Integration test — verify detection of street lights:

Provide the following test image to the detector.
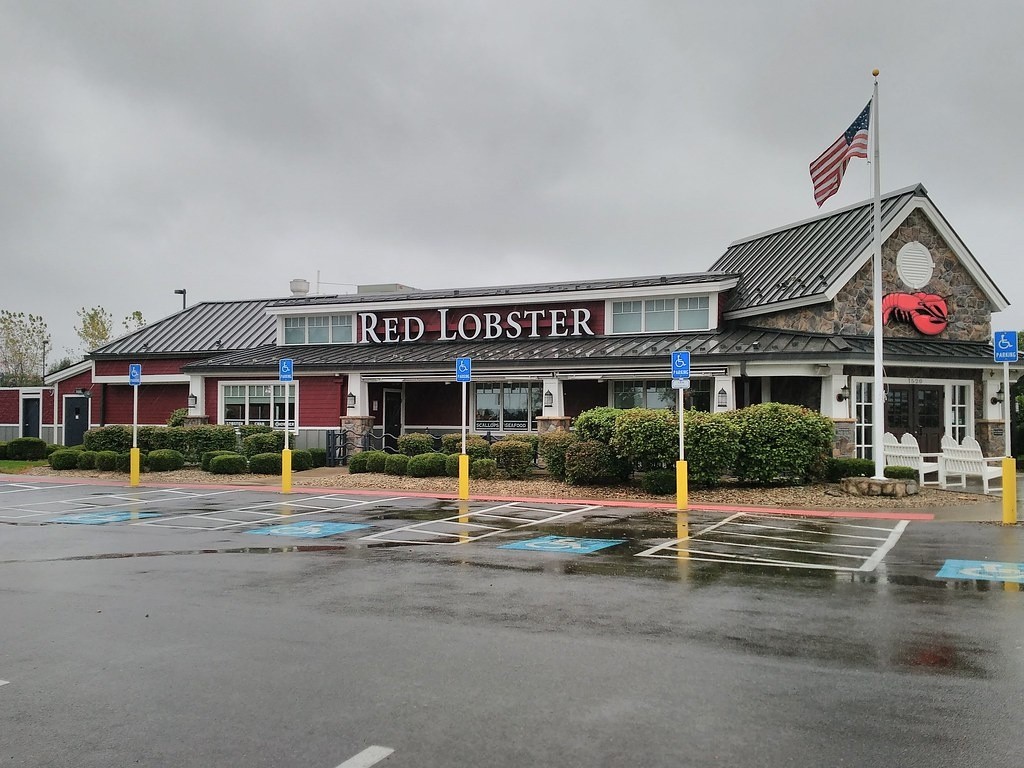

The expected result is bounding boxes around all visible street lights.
[174,289,186,309]
[42,340,50,377]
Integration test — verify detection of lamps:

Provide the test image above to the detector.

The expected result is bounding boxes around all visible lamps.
[187,393,197,408]
[836,385,850,403]
[991,389,1004,405]
[718,388,727,407]
[347,392,356,408]
[544,389,553,407]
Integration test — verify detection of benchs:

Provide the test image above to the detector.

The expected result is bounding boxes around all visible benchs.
[941,434,1007,495]
[884,432,941,487]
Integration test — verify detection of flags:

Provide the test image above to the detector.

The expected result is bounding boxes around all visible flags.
[809,96,872,208]
